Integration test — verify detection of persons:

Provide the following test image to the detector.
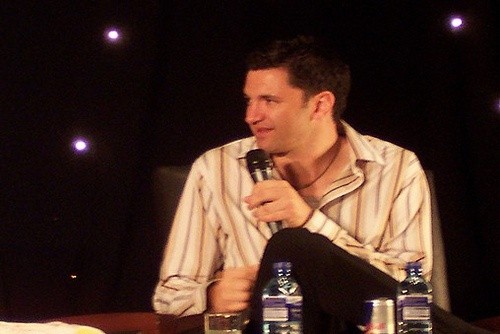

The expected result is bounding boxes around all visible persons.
[152,32,432,318]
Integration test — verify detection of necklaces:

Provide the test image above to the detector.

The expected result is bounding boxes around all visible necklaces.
[271,138,342,189]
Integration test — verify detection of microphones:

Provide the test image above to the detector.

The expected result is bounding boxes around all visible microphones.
[245,149,283,235]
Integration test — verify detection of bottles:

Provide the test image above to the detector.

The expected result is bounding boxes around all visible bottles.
[262,262,303,334]
[398,262,433,334]
